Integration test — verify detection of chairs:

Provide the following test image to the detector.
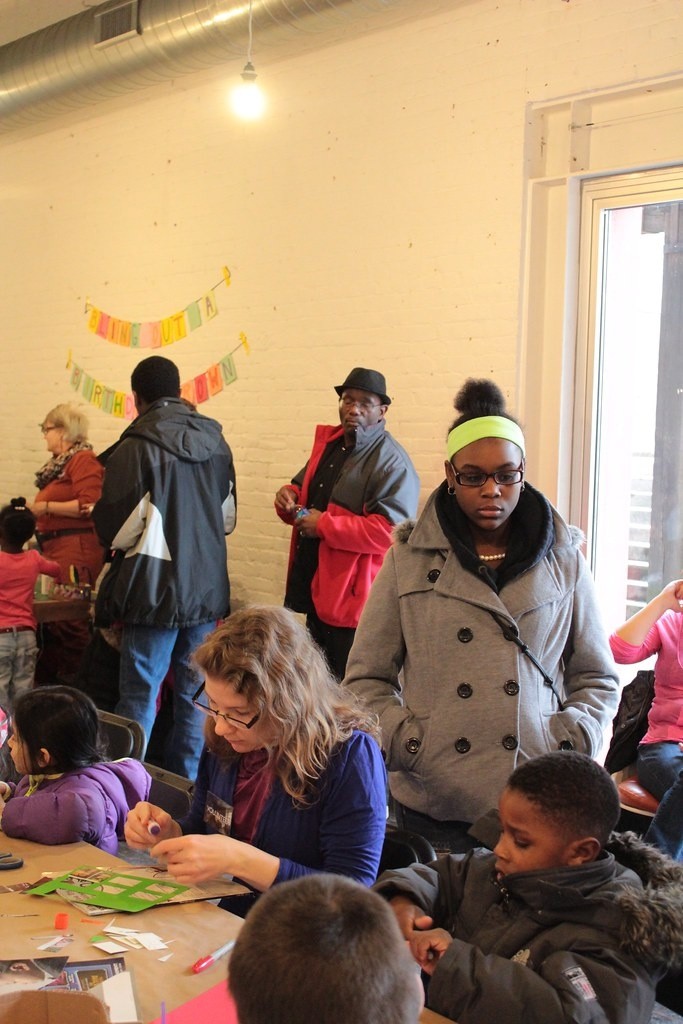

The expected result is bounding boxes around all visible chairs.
[374,826,439,888]
[615,670,658,827]
[135,760,197,828]
[95,711,145,762]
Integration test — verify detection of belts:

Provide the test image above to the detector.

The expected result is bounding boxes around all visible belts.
[36,527,95,541]
[0,626,34,633]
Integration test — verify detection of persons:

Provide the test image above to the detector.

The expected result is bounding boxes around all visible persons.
[228,872,424,1024]
[340,380,623,867]
[371,749,683,1023]
[0,497,59,786]
[273,369,420,686]
[0,685,151,855]
[24,404,106,689]
[123,606,387,922]
[90,357,236,832]
[608,580,683,866]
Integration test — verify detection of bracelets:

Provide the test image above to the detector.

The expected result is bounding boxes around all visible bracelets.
[45,501,48,515]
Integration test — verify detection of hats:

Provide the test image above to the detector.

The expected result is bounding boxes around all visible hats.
[333,367,391,406]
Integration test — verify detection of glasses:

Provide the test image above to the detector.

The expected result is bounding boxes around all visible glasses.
[448,458,525,487]
[339,396,382,411]
[191,680,262,729]
[41,426,64,435]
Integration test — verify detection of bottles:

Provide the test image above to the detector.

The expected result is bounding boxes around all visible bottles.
[289,502,309,524]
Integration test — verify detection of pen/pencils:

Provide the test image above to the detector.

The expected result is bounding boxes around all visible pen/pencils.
[191,940,236,974]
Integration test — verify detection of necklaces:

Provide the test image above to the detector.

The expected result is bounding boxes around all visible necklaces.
[478,553,505,561]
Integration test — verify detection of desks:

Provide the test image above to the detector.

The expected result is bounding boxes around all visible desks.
[2,824,455,1024]
[30,591,92,662]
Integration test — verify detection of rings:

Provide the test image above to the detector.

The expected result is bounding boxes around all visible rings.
[299,530,305,537]
